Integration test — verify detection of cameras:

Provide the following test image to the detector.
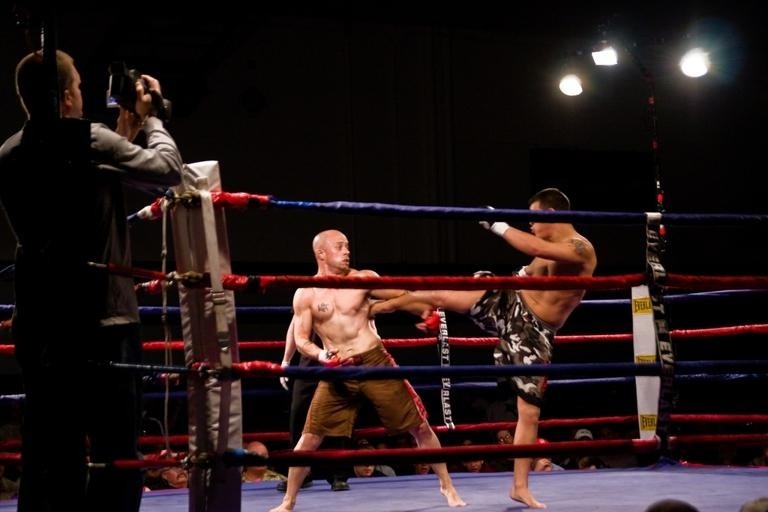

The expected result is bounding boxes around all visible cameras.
[103,68,173,118]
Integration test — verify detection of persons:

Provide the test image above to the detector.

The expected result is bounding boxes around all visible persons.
[645,497,698,511]
[276,312,352,491]
[266,229,470,512]
[1,429,611,495]
[366,185,599,509]
[739,495,768,512]
[0,45,185,512]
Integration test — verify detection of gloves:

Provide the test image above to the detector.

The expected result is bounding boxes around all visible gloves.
[280,360,290,391]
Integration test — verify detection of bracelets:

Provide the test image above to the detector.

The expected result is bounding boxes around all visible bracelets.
[489,220,510,237]
[518,264,528,278]
[318,349,328,364]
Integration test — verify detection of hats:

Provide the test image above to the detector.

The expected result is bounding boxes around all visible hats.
[575,428,594,440]
